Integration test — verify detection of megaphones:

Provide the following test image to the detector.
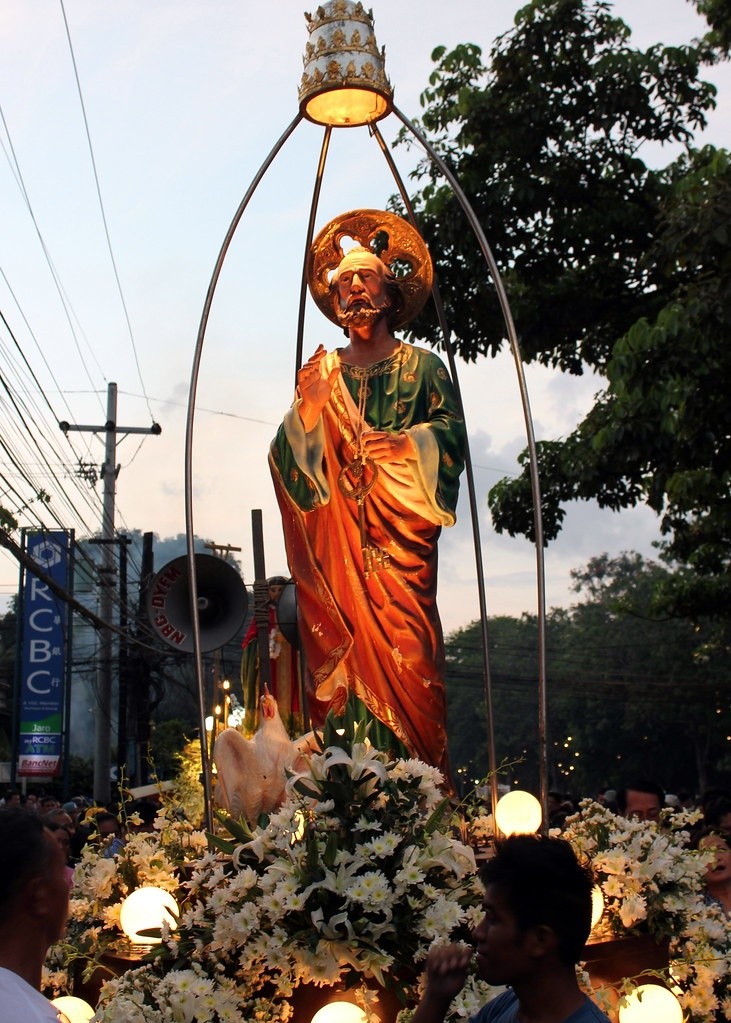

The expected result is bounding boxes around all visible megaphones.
[148,553,248,655]
[276,578,297,649]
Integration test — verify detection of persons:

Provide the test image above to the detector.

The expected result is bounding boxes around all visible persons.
[268,248,465,837]
[4,789,161,871]
[0,804,96,1023]
[549,780,731,922]
[240,577,286,730]
[411,834,612,1023]
